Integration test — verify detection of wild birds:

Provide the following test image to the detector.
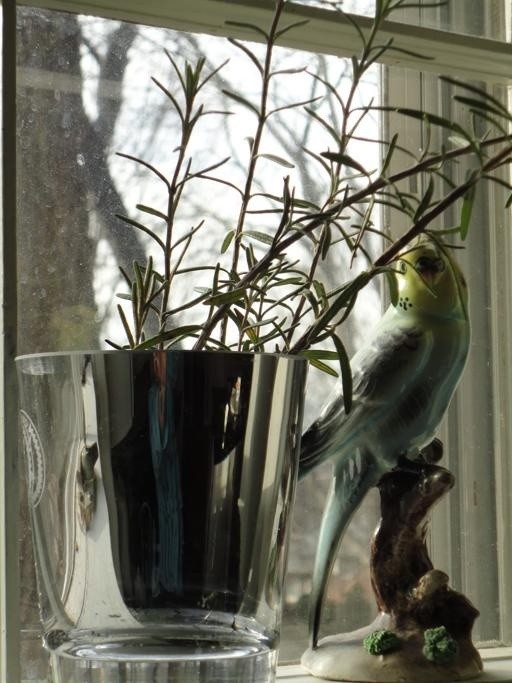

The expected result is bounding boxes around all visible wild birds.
[296,233,471,651]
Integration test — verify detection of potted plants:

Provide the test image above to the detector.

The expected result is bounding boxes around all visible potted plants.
[4,1,512,680]
